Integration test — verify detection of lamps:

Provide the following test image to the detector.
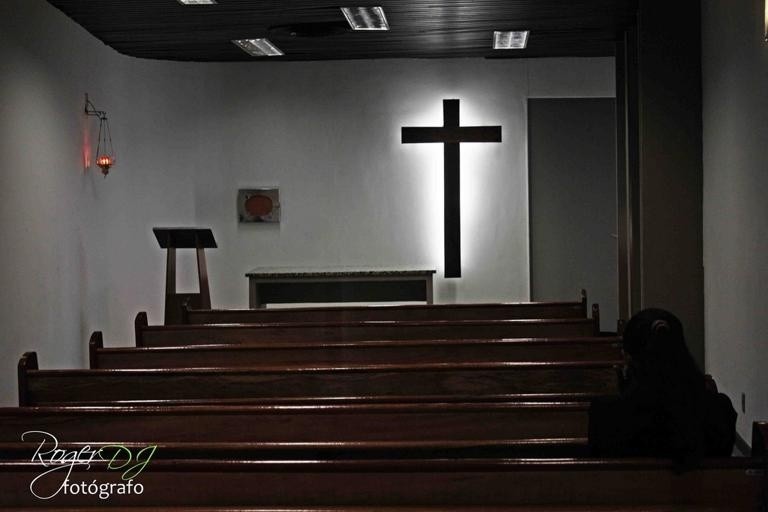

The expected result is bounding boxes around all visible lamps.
[80,89,117,180]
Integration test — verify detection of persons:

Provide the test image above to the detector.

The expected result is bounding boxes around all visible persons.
[588,307,738,464]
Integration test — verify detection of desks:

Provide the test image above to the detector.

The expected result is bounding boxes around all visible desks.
[245,268,436,311]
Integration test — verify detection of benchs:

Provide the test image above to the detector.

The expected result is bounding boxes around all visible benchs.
[0,288,763,507]
